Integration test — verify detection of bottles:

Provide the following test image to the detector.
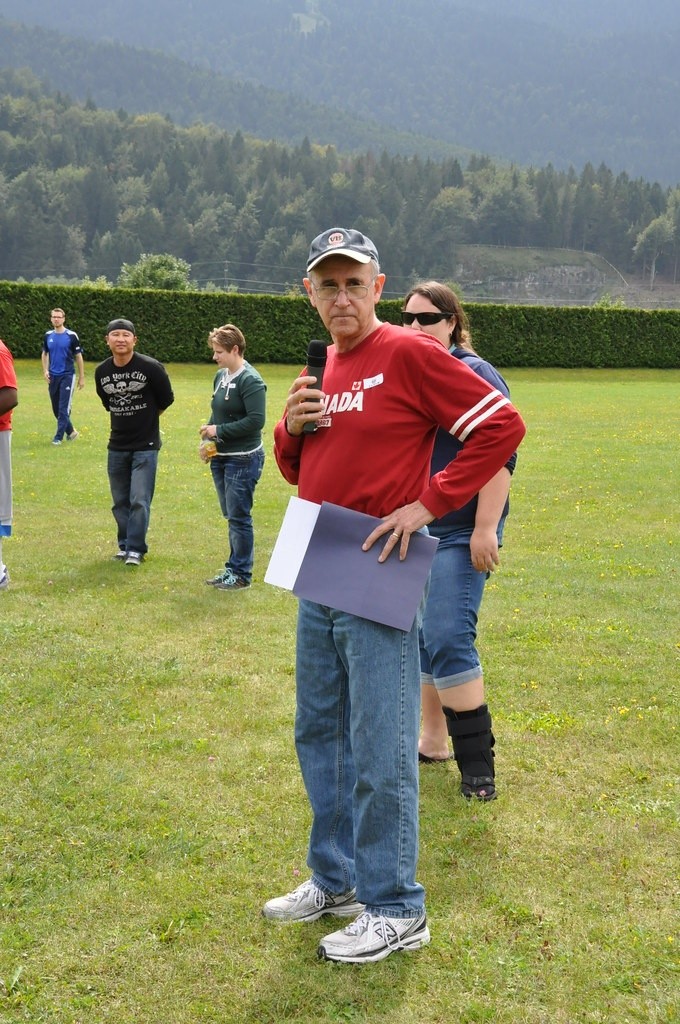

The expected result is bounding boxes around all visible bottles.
[200,419,217,457]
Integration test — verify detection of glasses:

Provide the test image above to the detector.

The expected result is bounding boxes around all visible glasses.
[51,315,66,320]
[401,310,455,325]
[308,278,377,301]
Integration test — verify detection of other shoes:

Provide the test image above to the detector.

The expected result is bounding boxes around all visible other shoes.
[67,429,78,441]
[52,438,61,444]
[0,564,9,589]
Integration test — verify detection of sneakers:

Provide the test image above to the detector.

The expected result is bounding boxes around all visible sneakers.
[318,907,431,964]
[262,876,368,924]
[215,571,251,592]
[116,551,126,561]
[126,553,141,566]
[206,569,232,585]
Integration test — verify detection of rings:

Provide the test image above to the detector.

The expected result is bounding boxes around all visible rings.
[393,533,400,538]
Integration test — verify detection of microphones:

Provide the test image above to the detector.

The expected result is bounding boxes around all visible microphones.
[303,340,327,433]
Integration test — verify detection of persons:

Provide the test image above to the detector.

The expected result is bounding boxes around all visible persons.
[94,319,175,566]
[260,223,525,961]
[400,281,517,802]
[0,338,18,589]
[41,308,85,445]
[198,323,267,592]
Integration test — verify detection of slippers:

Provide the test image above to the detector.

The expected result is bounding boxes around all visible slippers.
[416,752,454,763]
[457,771,498,801]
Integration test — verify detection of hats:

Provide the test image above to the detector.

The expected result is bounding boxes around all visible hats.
[107,319,135,336]
[305,227,379,274]
